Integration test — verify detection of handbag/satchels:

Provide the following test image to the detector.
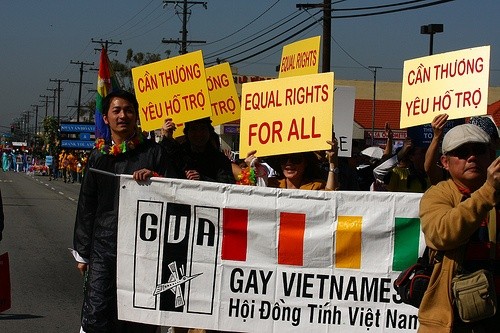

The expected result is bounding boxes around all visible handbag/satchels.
[450,268,496,324]
[394,264,432,309]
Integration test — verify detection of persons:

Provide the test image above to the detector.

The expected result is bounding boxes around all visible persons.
[158,114,500,193]
[417,124,500,333]
[67,90,166,333]
[0,140,89,183]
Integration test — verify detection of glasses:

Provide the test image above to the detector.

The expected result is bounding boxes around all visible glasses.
[280,155,305,166]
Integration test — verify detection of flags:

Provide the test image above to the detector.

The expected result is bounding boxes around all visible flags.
[95,48,112,142]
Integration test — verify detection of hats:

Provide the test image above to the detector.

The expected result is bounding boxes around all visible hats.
[441,124,492,155]
